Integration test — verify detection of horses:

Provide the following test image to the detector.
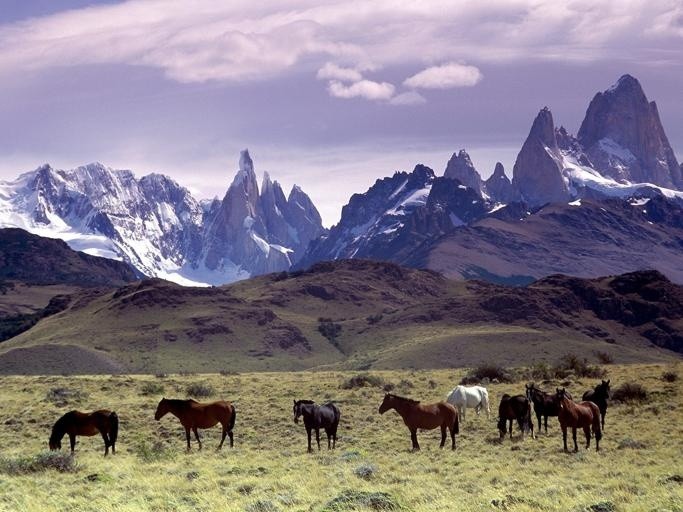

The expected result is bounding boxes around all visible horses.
[47,408,119,458]
[447,384,490,421]
[153,396,237,451]
[378,391,460,451]
[292,398,340,451]
[495,379,612,453]
